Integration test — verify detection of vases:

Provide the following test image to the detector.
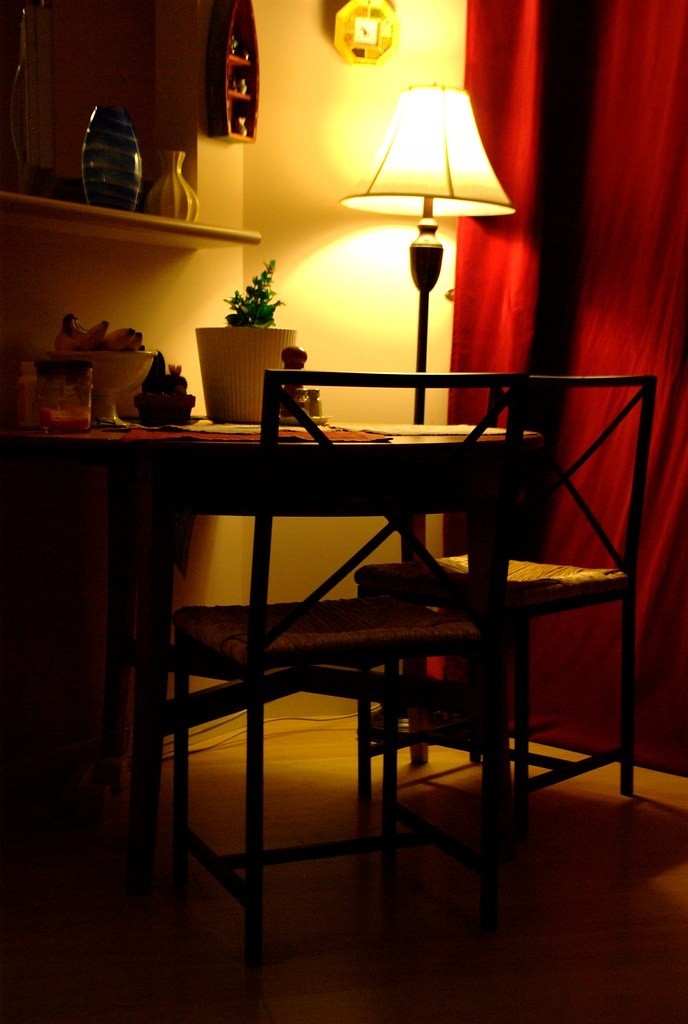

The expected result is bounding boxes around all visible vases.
[146,151,199,224]
[81,106,142,211]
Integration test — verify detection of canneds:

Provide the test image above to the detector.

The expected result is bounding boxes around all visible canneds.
[34,359,93,432]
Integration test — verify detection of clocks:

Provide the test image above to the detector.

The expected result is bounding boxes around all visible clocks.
[334,0,399,66]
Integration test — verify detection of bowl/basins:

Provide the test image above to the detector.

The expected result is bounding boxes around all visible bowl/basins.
[49,351,156,426]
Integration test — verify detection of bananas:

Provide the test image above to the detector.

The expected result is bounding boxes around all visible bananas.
[54,312,143,351]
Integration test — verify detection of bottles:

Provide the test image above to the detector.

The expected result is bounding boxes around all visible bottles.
[146,151,198,222]
[82,105,140,212]
[35,361,92,433]
[17,361,35,427]
[295,388,324,417]
[277,346,307,416]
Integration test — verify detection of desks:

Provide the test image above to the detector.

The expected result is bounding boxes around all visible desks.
[0,419,543,898]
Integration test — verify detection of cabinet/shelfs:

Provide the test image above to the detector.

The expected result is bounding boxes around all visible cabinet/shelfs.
[206,0,260,141]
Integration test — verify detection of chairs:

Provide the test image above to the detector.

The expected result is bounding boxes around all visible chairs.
[355,375,659,844]
[171,368,530,970]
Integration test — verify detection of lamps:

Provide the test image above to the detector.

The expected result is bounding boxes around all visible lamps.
[338,84,517,425]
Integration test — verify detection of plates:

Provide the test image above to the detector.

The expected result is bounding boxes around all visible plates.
[278,416,330,431]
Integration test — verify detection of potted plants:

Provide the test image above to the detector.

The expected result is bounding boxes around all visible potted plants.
[195,260,298,424]
